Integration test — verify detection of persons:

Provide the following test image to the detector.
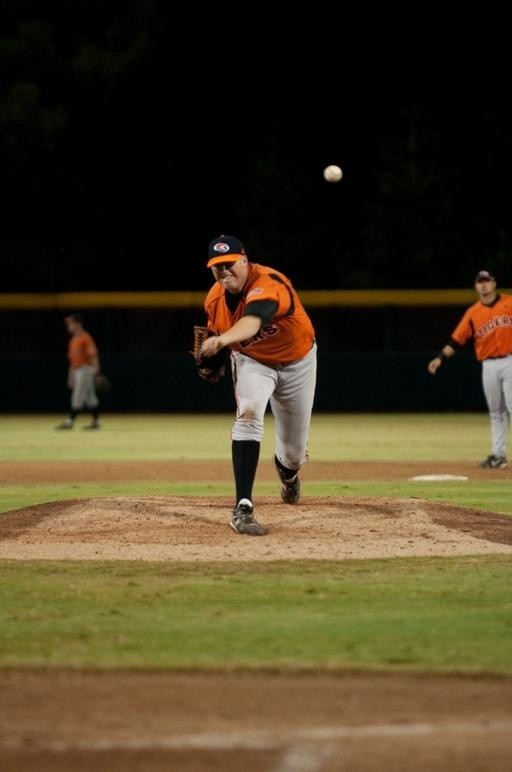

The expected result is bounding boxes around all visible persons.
[56,315,103,429]
[199,236,317,536]
[428,270,512,469]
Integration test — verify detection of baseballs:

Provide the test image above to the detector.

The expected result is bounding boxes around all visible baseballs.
[324,165,342,181]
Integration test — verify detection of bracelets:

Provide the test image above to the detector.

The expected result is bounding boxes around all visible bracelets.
[438,352,448,362]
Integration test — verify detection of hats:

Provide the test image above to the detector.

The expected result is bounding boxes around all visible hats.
[206,234,245,268]
[475,270,495,281]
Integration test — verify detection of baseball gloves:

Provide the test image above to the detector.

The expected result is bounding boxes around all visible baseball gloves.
[193,326,232,383]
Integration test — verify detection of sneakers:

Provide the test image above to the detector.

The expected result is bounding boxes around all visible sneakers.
[274,456,300,504]
[480,455,507,470]
[229,497,264,535]
